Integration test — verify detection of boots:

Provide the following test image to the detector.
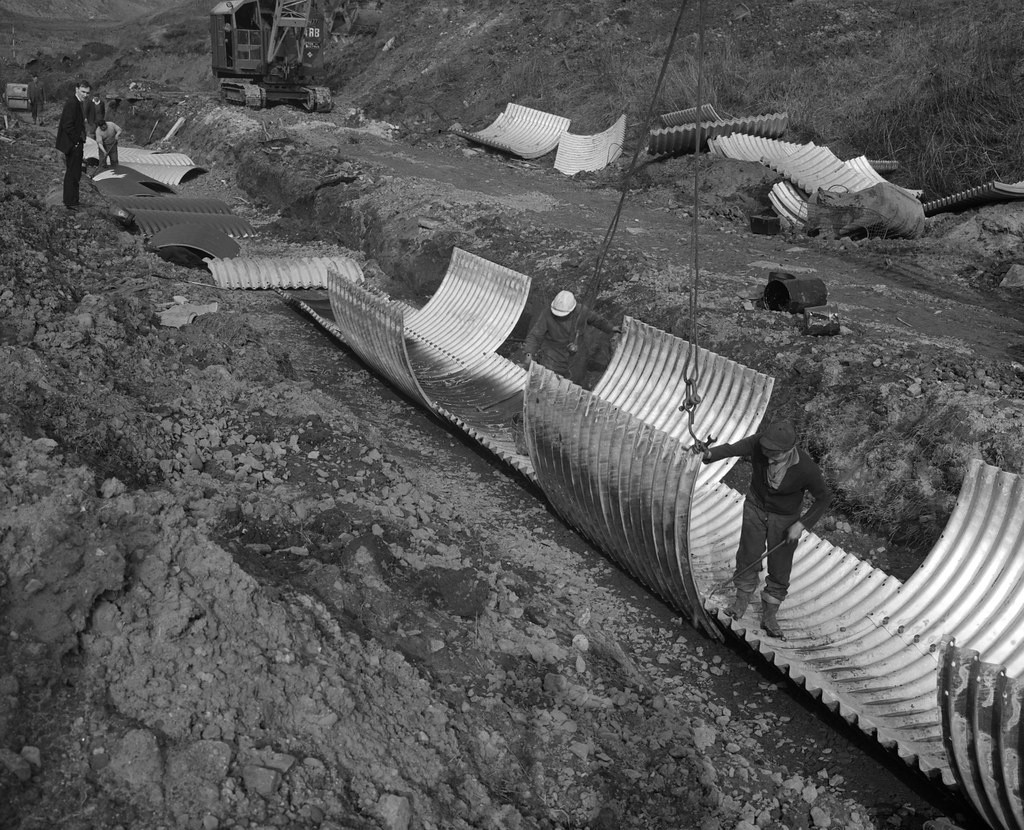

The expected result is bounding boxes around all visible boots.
[38,117,44,126]
[759,597,784,636]
[723,589,755,621]
[33,117,36,125]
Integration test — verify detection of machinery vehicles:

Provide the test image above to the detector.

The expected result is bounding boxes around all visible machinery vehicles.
[209,0,334,115]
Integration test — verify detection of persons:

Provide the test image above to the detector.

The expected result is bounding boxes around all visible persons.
[690,418,832,639]
[95,120,123,168]
[521,289,622,378]
[84,92,106,140]
[56,80,92,213]
[27,73,47,125]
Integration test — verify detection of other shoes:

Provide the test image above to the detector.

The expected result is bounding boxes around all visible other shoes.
[66,204,85,212]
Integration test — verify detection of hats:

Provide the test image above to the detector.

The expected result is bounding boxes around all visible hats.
[758,422,796,450]
[550,290,577,317]
